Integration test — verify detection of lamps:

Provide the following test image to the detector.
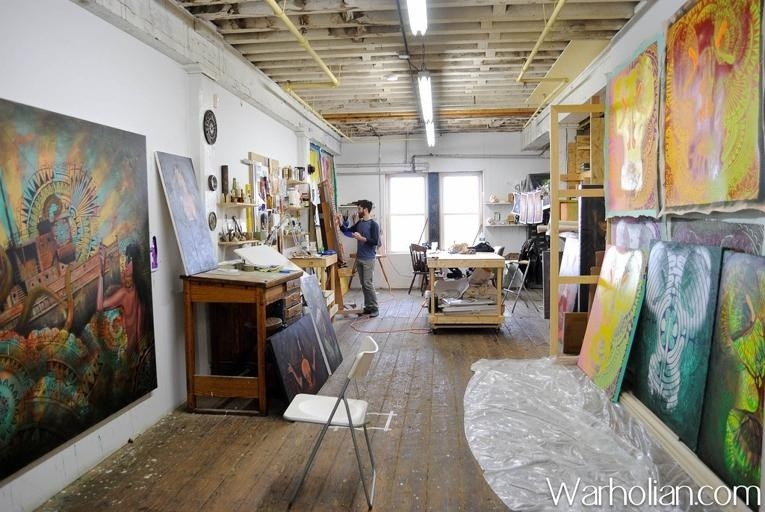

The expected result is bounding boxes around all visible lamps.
[404,0,428,39]
[425,123,436,148]
[416,62,433,123]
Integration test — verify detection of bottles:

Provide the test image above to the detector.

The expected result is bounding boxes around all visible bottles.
[288,165,304,181]
[301,192,309,207]
[230,178,239,202]
[266,194,273,209]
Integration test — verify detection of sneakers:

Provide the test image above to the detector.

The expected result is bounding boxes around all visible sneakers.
[356,308,379,317]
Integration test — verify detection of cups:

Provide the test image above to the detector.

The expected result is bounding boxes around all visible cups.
[244,264,255,272]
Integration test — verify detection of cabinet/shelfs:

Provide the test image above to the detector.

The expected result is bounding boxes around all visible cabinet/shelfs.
[217,201,260,249]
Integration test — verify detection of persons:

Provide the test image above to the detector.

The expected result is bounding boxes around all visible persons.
[288,346,322,394]
[337,200,379,317]
[96,245,154,368]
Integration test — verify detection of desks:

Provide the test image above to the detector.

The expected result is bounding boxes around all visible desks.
[426,249,505,330]
[178,266,303,416]
[289,253,339,324]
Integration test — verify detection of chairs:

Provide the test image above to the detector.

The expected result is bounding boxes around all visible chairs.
[408,242,442,294]
[283,335,379,510]
[499,238,541,313]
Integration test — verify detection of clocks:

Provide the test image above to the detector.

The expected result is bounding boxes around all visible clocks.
[203,110,218,146]
